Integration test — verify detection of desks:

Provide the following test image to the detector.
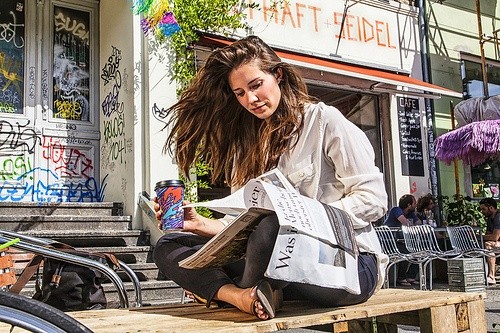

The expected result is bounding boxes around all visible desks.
[0,288,489,333]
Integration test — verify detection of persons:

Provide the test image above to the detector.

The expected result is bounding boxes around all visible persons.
[475,197,500,285]
[153,33,389,320]
[415,193,443,239]
[381,195,424,286]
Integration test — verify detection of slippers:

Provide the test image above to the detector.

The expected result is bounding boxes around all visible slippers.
[250,280,276,320]
[409,279,421,285]
[185,290,217,306]
[400,281,413,287]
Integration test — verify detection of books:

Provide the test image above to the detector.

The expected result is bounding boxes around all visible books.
[176,168,363,295]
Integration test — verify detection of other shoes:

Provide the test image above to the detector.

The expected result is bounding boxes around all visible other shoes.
[487,276,497,284]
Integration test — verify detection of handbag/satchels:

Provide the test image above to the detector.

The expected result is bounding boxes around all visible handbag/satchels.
[9,243,120,313]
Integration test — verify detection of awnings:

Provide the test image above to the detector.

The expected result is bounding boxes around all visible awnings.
[194,30,465,100]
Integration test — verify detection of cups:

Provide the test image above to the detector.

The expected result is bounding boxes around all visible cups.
[154,179,185,230]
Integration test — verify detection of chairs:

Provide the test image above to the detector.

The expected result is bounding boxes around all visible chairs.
[376,225,492,288]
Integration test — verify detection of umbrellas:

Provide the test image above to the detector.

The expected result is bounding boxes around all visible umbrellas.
[434,95,500,166]
